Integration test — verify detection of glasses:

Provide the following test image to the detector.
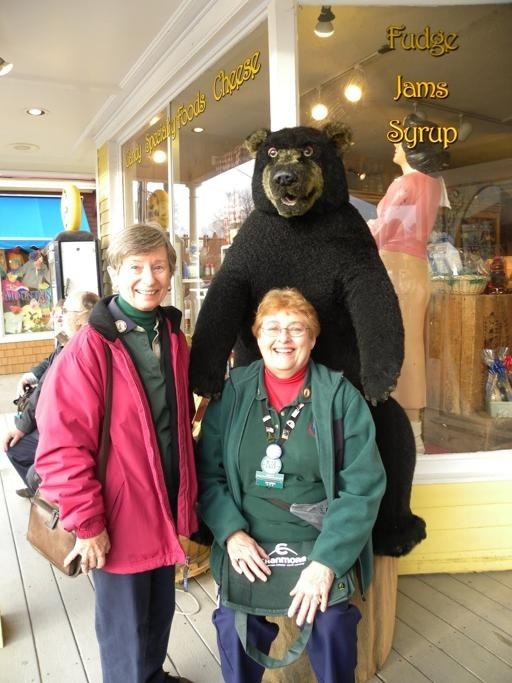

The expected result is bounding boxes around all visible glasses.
[258,323,311,337]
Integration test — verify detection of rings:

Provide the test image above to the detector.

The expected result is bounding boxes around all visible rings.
[235,558,244,563]
[313,593,321,604]
[79,561,88,565]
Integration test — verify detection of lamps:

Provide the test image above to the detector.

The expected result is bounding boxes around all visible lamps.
[0,57,14,76]
[312,6,337,39]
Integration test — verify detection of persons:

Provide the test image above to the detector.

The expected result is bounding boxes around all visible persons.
[9,249,51,290]
[34,224,200,682]
[366,113,445,455]
[3,289,107,499]
[196,289,391,682]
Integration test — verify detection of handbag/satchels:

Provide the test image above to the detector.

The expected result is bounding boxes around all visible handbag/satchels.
[14,384,36,412]
[289,498,328,533]
[220,541,355,615]
[26,488,82,577]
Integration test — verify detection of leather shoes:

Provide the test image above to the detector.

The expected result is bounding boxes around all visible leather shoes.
[17,489,36,498]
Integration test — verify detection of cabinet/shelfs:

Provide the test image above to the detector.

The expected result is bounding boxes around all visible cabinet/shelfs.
[423,292,512,417]
[421,408,512,452]
